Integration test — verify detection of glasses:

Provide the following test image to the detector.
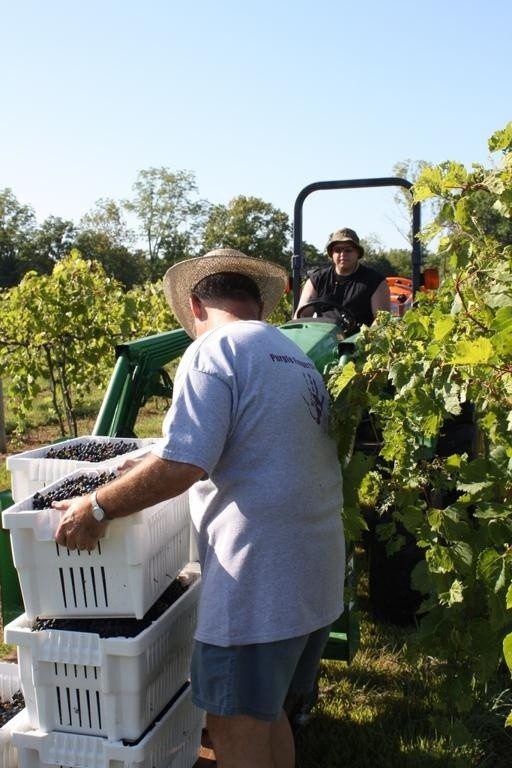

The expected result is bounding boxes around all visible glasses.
[332,246,354,252]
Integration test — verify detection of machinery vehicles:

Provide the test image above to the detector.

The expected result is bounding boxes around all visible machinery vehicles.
[1,173,480,667]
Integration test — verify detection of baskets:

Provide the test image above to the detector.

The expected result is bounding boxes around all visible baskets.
[0,435,204,767]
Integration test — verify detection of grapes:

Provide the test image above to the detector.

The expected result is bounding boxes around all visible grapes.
[0,439,192,730]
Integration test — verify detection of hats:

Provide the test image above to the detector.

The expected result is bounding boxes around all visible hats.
[163,248,289,342]
[326,228,363,259]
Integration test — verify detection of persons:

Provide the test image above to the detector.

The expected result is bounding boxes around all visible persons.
[53,248,345,767]
[290,226,391,347]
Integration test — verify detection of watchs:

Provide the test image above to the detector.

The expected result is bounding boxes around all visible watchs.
[91,490,110,523]
